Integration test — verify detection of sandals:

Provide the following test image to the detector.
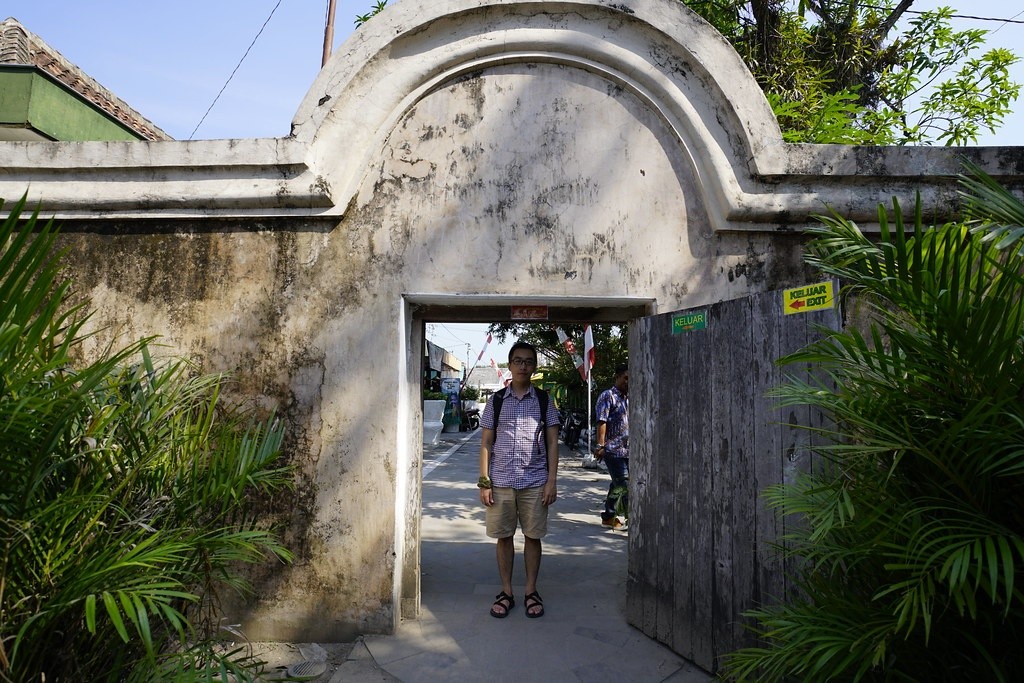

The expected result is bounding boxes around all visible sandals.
[490,591,515,618]
[524,591,544,618]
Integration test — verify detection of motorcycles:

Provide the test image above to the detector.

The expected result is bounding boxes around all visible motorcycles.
[565,408,588,449]
[463,407,482,430]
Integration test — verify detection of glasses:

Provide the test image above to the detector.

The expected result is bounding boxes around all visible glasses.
[511,359,535,366]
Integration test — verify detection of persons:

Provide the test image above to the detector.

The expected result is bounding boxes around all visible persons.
[476,342,561,618]
[594,365,628,531]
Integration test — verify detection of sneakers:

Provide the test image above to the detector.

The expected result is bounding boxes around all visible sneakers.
[601,517,628,530]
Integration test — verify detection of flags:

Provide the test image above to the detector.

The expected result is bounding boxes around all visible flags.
[584,323,595,378]
